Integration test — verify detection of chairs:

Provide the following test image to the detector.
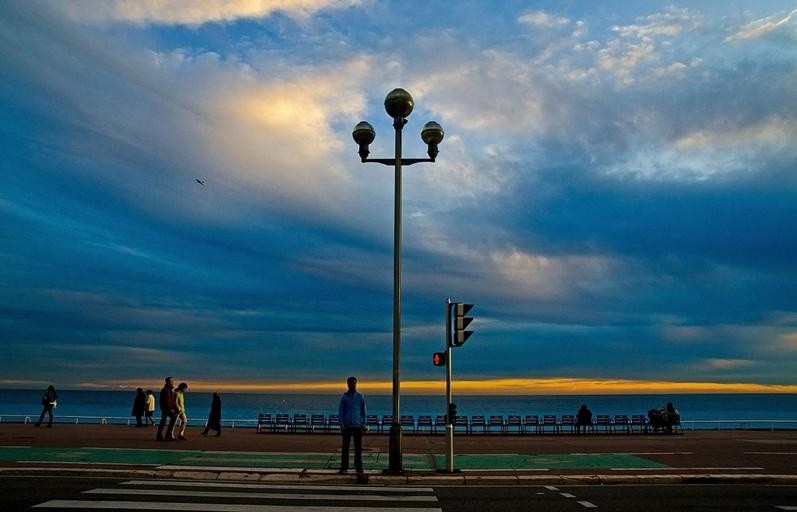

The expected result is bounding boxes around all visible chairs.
[255,410,684,433]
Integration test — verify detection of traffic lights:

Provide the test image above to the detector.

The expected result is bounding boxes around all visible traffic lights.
[448,402,458,426]
[433,351,447,368]
[453,301,476,346]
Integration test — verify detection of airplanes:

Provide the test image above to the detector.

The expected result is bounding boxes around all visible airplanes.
[194,178,204,186]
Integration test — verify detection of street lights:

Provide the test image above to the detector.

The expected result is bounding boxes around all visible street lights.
[351,87,447,474]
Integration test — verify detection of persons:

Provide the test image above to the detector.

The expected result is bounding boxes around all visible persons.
[200,392,223,438]
[648,401,681,433]
[131,375,188,440]
[338,377,371,483]
[576,404,595,434]
[35,386,57,430]
